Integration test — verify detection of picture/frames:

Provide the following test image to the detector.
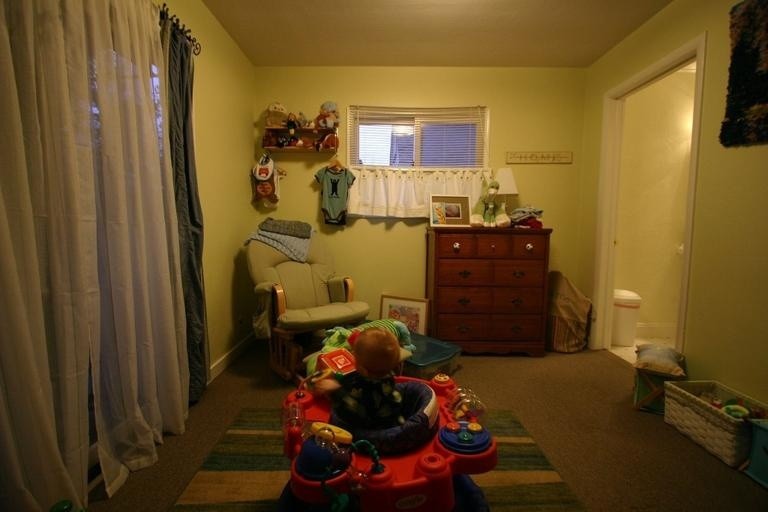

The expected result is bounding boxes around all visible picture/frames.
[379,294,431,337]
[429,193,473,228]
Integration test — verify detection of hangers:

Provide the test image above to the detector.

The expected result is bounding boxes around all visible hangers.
[327,156,343,171]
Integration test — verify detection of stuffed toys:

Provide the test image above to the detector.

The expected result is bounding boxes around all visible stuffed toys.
[276,99,338,151]
[471,172,512,228]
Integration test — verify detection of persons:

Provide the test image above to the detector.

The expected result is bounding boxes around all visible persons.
[314,317,412,437]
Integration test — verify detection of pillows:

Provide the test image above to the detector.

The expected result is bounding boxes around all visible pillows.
[633,340,688,378]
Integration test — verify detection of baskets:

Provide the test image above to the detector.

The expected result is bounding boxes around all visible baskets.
[662,378,768,467]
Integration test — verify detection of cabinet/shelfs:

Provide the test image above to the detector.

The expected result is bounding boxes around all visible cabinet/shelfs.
[263,125,338,152]
[425,228,554,357]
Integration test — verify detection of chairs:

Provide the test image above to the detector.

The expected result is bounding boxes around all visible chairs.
[244,233,369,388]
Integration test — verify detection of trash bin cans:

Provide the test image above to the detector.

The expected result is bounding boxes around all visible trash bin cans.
[612,288,642,346]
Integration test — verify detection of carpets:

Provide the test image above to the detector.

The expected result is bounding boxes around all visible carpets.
[167,407,584,512]
